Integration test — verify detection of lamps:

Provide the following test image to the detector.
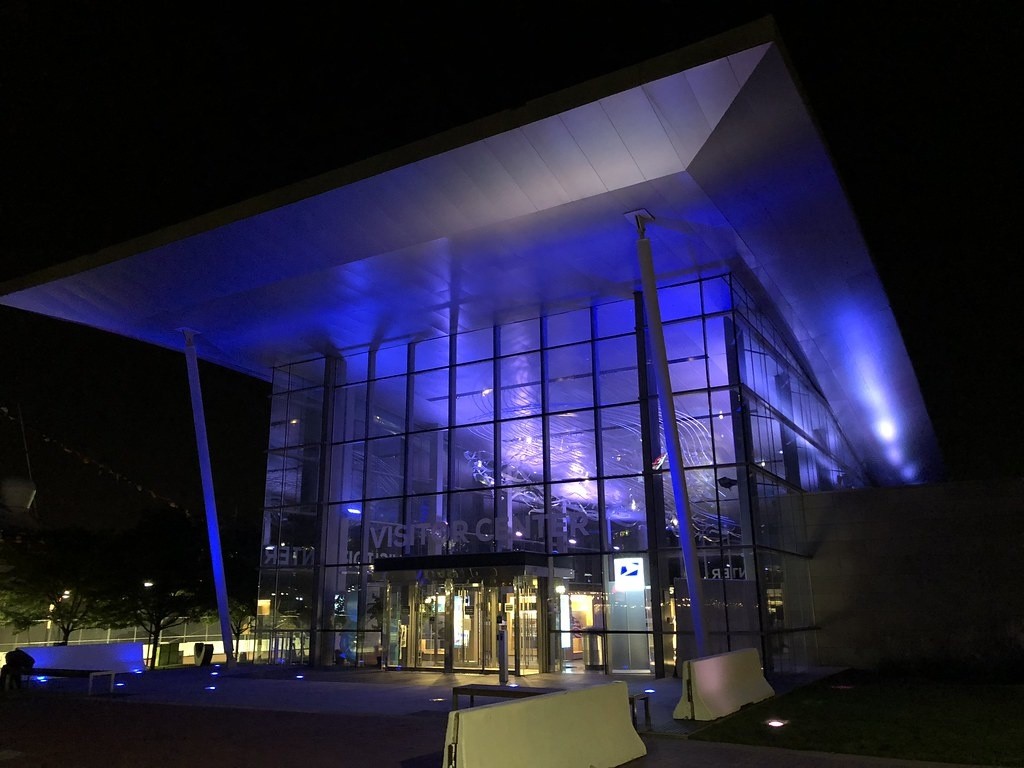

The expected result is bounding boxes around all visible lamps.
[718,477,738,488]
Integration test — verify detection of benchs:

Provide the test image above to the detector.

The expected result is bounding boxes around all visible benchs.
[453,684,652,734]
[4,666,115,696]
